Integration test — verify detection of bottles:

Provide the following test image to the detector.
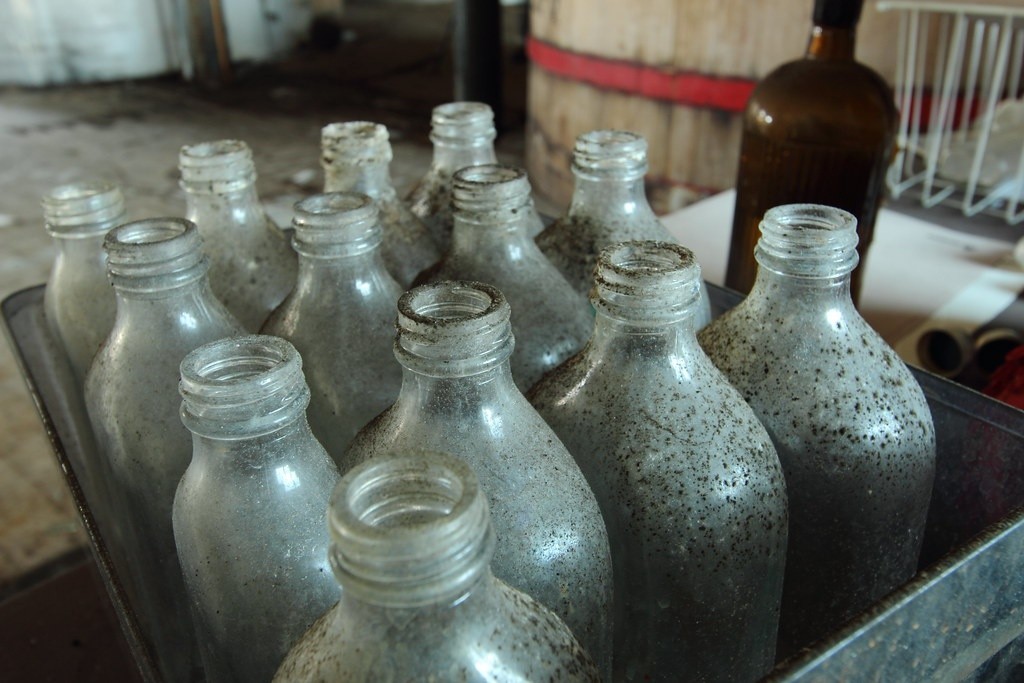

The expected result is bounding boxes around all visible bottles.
[39,0,938,683]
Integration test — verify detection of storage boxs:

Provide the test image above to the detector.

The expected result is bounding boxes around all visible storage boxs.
[1,213,1024,683]
